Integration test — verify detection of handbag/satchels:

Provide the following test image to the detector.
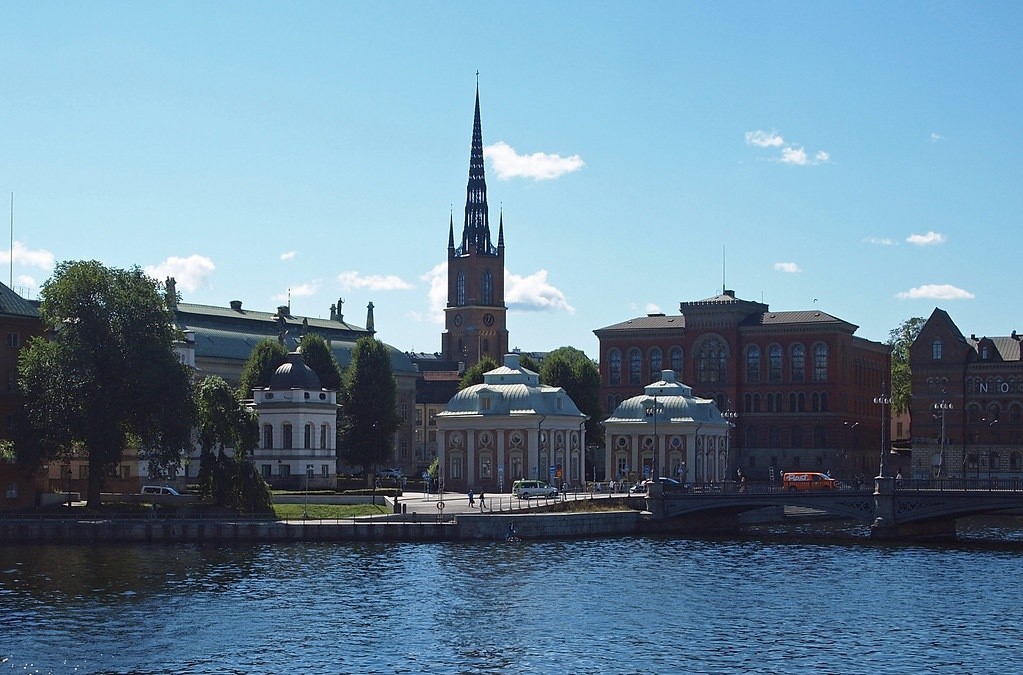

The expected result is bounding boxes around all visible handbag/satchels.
[472,500,475,503]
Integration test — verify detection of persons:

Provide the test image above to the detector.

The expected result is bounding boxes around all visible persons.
[609,478,614,493]
[468,490,474,508]
[479,490,486,508]
[896,471,902,488]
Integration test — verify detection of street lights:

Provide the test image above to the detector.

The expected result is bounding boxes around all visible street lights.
[375,468,403,478]
[844,421,861,473]
[386,458,389,478]
[439,482,444,523]
[981,418,999,491]
[873,382,894,477]
[836,447,848,475]
[934,388,954,480]
[67,468,73,507]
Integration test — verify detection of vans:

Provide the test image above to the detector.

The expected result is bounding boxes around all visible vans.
[784,472,835,492]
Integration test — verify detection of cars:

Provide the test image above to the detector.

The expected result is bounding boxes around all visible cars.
[141,485,181,496]
[629,486,637,493]
[512,481,520,487]
[351,471,364,478]
[420,473,430,481]
[643,477,688,493]
[513,480,558,499]
[639,480,646,494]
[836,482,852,489]
